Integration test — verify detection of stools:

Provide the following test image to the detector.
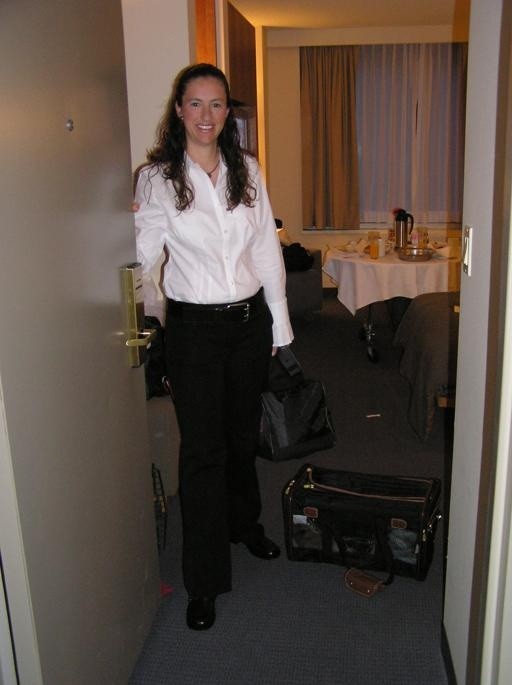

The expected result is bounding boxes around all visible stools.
[393,292,460,442]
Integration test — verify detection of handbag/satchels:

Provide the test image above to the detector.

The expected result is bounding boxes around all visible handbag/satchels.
[259,346,338,459]
[143,352,178,403]
[282,462,442,581]
[283,243,314,272]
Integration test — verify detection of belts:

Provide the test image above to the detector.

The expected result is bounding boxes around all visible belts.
[169,288,265,323]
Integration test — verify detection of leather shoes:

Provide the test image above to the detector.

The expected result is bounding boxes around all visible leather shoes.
[228,524,280,560]
[187,586,216,631]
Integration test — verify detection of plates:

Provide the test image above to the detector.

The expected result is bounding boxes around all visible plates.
[396,247,435,261]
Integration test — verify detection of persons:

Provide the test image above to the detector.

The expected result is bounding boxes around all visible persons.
[133,62,297,631]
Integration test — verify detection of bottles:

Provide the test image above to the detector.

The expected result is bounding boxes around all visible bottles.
[376,237,385,258]
[387,228,396,255]
[395,209,414,251]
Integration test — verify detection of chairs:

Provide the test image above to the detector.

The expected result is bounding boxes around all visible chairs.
[286,248,322,323]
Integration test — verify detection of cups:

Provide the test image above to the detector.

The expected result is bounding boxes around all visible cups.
[367,231,380,242]
[416,226,428,249]
[370,241,380,260]
[409,231,418,245]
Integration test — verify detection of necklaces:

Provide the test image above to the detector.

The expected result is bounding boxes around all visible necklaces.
[207,159,220,178]
